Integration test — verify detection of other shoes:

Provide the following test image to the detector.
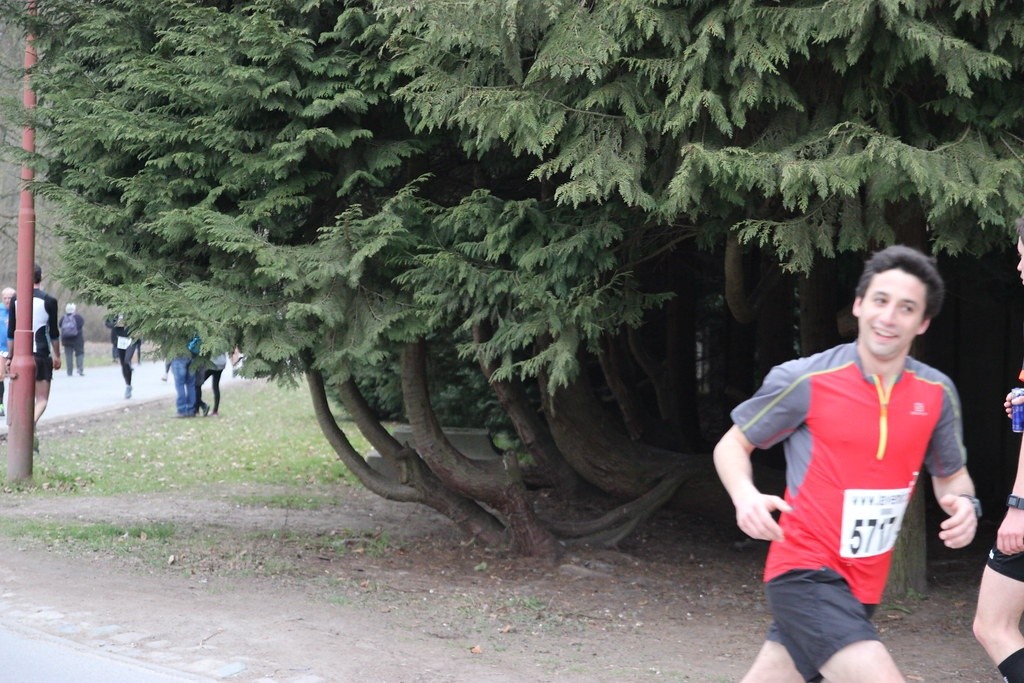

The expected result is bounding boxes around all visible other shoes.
[208,412,219,416]
[202,402,210,416]
[124,384,132,399]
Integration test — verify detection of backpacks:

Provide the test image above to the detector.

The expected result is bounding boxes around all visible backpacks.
[61,314,78,338]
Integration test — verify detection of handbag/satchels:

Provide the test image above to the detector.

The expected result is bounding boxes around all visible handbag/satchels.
[116,336,133,350]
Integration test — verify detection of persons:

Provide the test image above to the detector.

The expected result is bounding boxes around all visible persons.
[972,220,1024,683]
[713,247,976,683]
[1,261,242,441]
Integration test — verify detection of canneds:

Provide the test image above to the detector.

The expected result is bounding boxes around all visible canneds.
[1011,388,1024,433]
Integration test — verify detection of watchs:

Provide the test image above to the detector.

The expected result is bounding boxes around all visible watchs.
[1006,494,1024,510]
[962,493,984,517]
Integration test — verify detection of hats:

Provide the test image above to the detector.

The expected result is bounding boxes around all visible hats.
[66,303,76,314]
